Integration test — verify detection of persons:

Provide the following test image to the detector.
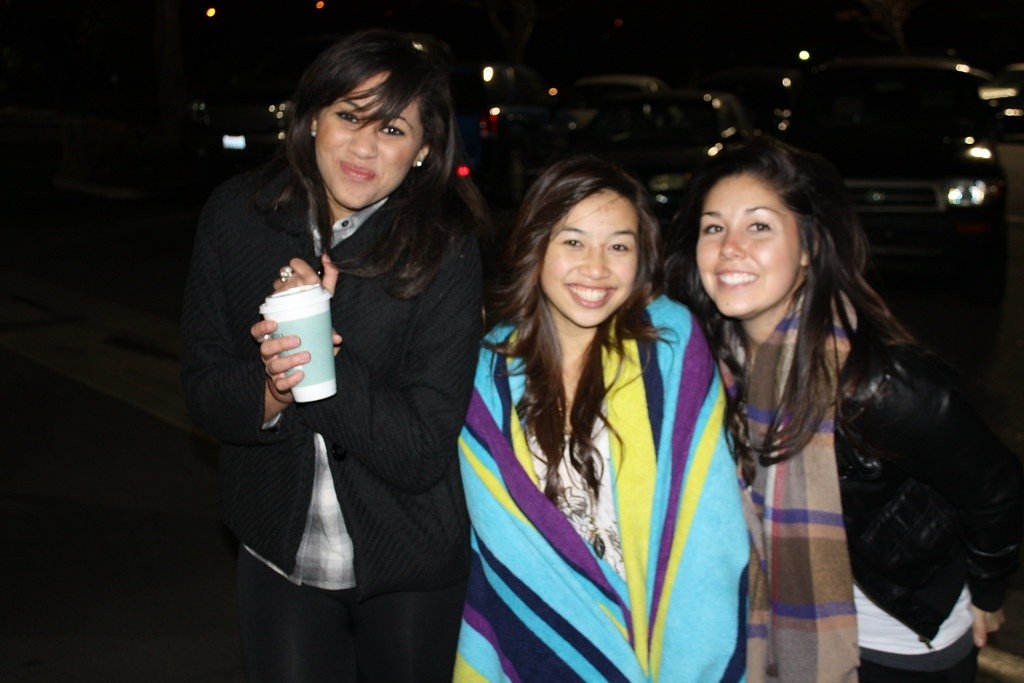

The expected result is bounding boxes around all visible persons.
[182,38,502,683]
[647,128,1024,683]
[452,159,749,683]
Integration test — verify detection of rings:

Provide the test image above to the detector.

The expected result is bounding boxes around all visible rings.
[281,265,293,282]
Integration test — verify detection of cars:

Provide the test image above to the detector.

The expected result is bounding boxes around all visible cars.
[990,63,1024,117]
[472,75,758,231]
[768,56,1020,313]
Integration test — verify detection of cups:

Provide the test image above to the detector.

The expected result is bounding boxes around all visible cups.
[258,282,336,404]
